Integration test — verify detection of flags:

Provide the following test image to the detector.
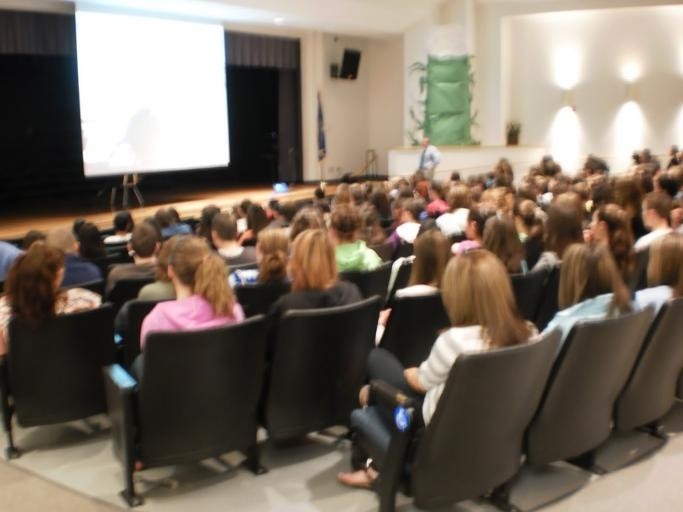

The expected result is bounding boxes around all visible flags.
[316,92,326,162]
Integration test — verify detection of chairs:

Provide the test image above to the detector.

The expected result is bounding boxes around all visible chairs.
[2,193,680,512]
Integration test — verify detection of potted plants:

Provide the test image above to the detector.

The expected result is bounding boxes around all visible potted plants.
[503,118,522,146]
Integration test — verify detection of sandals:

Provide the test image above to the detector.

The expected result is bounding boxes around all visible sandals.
[339,467,376,488]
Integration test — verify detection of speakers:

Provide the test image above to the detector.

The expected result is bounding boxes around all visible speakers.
[338,49,361,80]
